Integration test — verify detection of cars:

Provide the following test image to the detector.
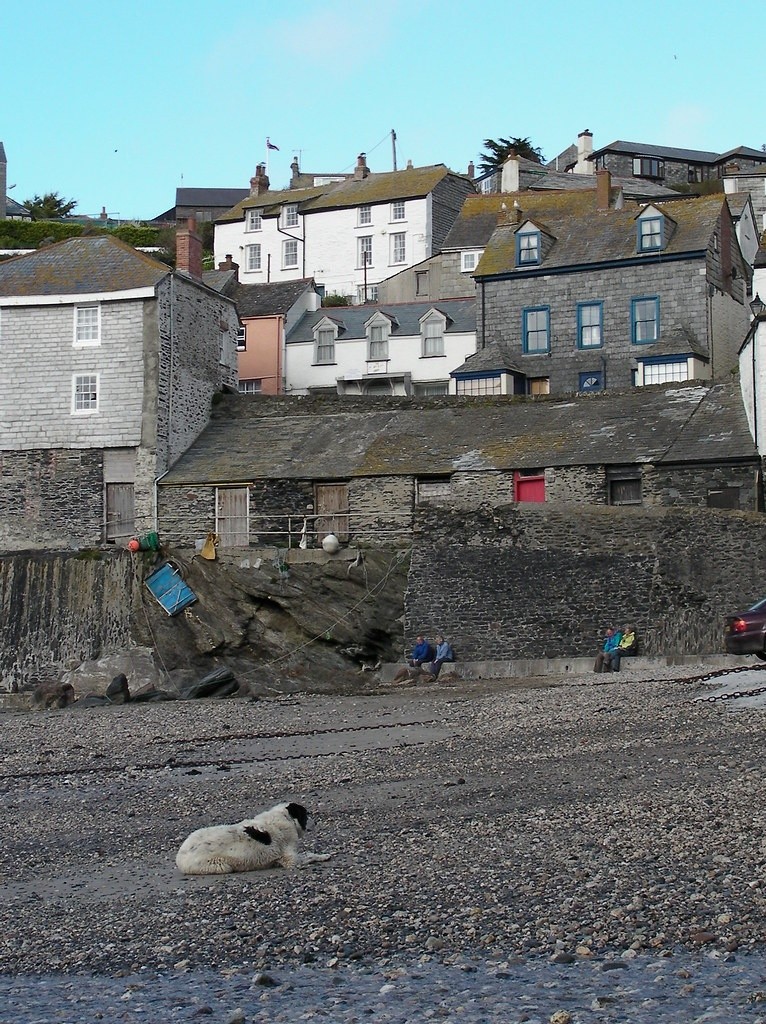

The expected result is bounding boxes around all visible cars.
[722,597,766,663]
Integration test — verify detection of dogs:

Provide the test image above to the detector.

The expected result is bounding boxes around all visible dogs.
[176,802,332,875]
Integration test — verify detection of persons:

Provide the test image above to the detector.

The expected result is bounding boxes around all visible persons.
[408,635,435,667]
[426,634,454,683]
[593,628,623,673]
[603,623,638,672]
